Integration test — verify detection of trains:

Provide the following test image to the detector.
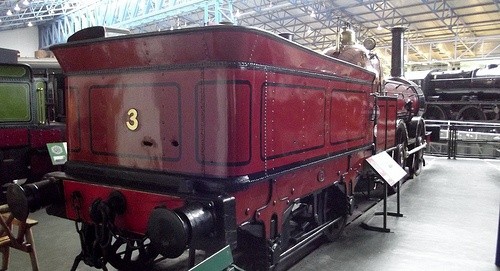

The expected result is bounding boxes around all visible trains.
[408,66,500,142]
[0,47,67,206]
[6,20,423,271]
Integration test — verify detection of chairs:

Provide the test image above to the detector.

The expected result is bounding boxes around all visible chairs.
[0,211,40,271]
